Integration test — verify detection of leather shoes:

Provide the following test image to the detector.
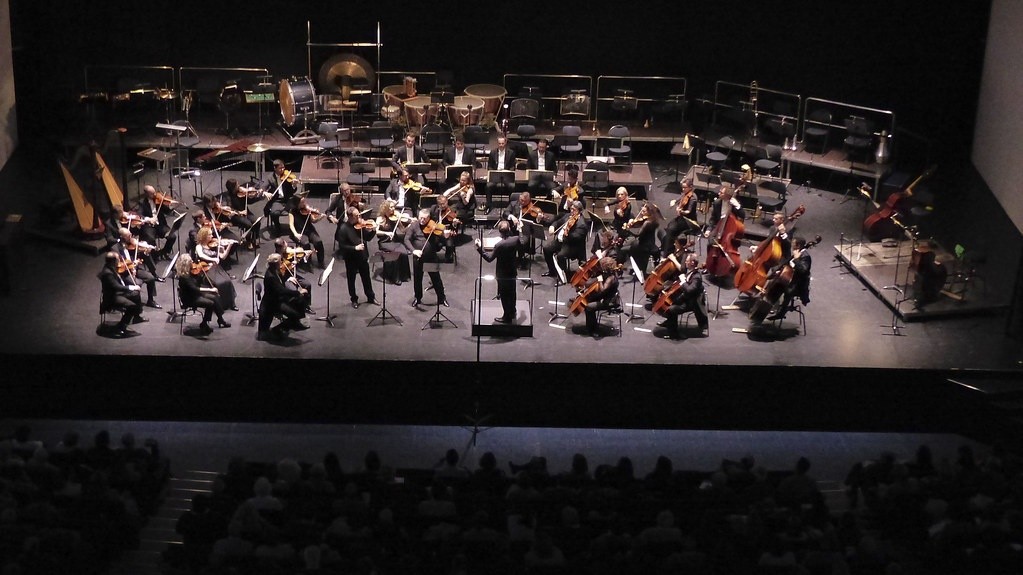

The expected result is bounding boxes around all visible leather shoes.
[412,299,420,307]
[368,299,380,305]
[352,301,358,308]
[443,300,450,307]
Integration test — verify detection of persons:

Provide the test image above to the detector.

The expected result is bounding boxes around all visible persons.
[99,132,811,341]
[0,424,1023,575]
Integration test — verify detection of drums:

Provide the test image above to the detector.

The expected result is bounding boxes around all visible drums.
[279,76,316,127]
[380,75,509,124]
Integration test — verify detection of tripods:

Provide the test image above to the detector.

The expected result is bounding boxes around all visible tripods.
[242,214,268,326]
[624,256,645,323]
[707,239,735,320]
[367,251,404,328]
[548,254,569,324]
[163,251,187,323]
[845,186,882,261]
[695,172,722,237]
[137,90,199,210]
[315,257,338,327]
[487,171,515,236]
[883,216,918,296]
[520,220,546,290]
[422,263,458,330]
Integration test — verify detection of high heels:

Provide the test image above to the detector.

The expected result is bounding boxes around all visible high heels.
[199,323,214,333]
[217,319,231,328]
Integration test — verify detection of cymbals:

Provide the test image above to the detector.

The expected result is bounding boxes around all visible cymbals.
[246,142,271,153]
[318,50,378,95]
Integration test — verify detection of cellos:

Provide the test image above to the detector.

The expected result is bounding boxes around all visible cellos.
[729,205,805,307]
[557,263,623,326]
[559,238,622,300]
[706,177,744,312]
[910,224,948,308]
[746,235,823,331]
[637,264,707,328]
[863,174,925,243]
[634,240,696,304]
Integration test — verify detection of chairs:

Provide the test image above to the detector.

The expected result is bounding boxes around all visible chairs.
[79,83,873,336]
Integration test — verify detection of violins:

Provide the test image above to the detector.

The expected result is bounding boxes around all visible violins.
[119,211,159,227]
[128,241,160,252]
[279,260,292,274]
[562,189,693,237]
[389,210,417,223]
[287,247,318,259]
[214,201,239,216]
[204,219,233,228]
[237,187,265,198]
[117,258,144,273]
[208,238,238,247]
[191,262,209,274]
[300,206,319,220]
[154,191,183,205]
[280,170,296,182]
[564,186,580,201]
[522,202,550,220]
[354,218,377,229]
[422,183,475,237]
[403,179,430,191]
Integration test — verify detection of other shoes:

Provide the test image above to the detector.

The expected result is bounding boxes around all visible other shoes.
[147,300,162,308]
[394,279,402,285]
[542,272,555,276]
[664,333,671,338]
[132,316,149,324]
[308,266,314,274]
[494,317,512,322]
[555,281,566,287]
[307,308,317,315]
[592,330,602,339]
[767,314,783,321]
[231,306,239,311]
[154,276,166,282]
[484,208,492,215]
[321,264,327,269]
[296,322,310,331]
[657,321,667,326]
